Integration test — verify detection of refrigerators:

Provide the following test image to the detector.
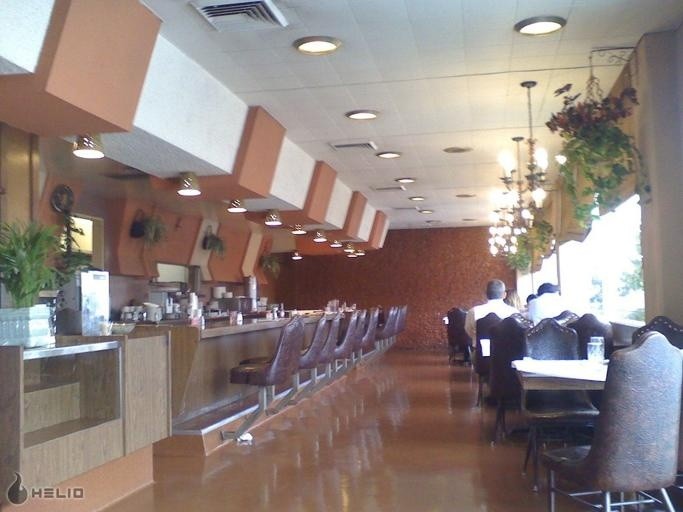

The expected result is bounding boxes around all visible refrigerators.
[57,270,110,337]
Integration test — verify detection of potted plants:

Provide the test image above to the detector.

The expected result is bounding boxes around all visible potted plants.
[3,219,87,347]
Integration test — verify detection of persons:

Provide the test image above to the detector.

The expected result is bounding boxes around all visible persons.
[524,294,536,308]
[463,279,519,380]
[526,283,567,327]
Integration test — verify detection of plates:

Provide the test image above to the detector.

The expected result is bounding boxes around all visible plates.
[213,286,233,299]
[120,304,143,321]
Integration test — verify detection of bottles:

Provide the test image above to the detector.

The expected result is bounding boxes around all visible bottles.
[236,311,243,322]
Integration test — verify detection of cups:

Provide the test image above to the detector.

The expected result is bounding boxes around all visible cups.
[587,336,605,368]
[101,321,111,336]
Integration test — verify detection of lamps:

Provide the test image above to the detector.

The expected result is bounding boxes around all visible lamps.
[378,152,435,216]
[513,17,566,37]
[73,132,104,160]
[177,173,201,197]
[345,110,380,121]
[228,198,365,261]
[490,80,569,258]
[293,36,341,54]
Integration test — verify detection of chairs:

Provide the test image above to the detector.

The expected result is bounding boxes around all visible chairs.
[220,306,408,448]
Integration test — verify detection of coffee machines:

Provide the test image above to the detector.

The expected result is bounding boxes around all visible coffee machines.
[147,281,190,320]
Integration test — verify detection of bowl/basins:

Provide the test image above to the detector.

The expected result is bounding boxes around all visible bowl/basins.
[110,323,137,334]
